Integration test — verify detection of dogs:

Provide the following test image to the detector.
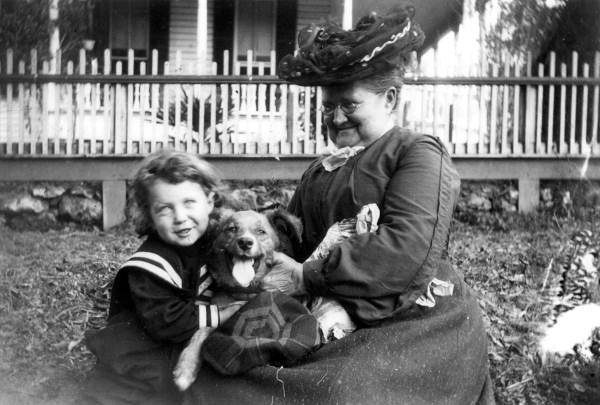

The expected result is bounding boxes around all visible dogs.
[172,206,355,392]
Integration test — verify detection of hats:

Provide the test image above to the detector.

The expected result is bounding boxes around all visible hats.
[278,4,426,86]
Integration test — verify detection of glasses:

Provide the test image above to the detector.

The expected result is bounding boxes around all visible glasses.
[318,92,384,114]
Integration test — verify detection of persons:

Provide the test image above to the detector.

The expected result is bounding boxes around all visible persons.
[181,7,495,405]
[84,147,247,405]
